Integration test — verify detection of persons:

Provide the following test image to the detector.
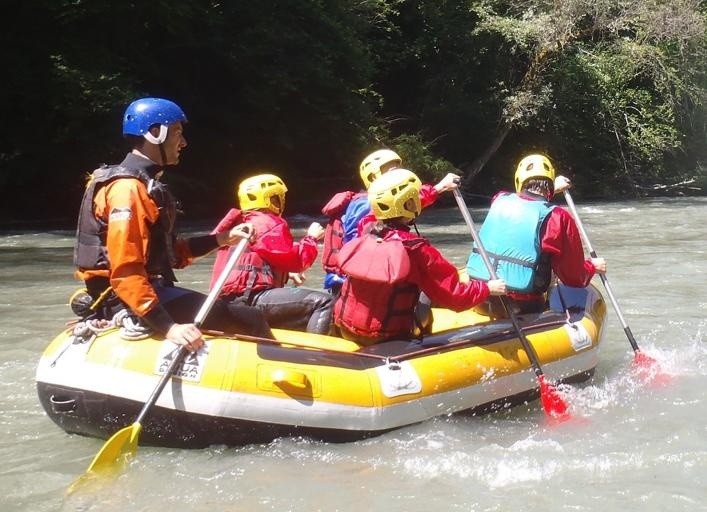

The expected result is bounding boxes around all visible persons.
[209,174,335,336]
[321,150,402,296]
[333,169,506,346]
[466,155,607,318]
[73,98,281,355]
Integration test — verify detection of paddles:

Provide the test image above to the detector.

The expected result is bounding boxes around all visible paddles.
[560,179,674,384]
[447,176,577,425]
[88,227,255,468]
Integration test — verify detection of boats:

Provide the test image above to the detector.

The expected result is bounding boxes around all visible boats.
[37,271,606,448]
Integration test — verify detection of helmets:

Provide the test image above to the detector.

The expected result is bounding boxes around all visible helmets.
[239,175,288,214]
[368,169,423,221]
[359,150,402,189]
[124,98,186,145]
[515,154,555,192]
